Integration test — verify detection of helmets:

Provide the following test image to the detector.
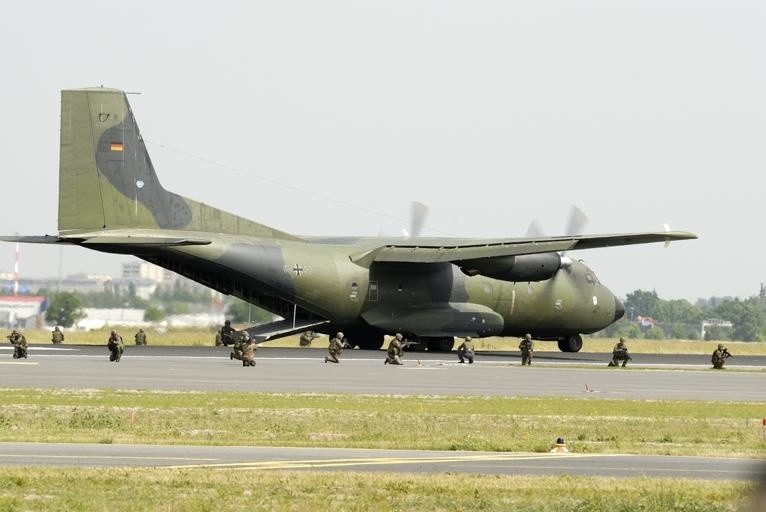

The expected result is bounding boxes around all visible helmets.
[620,337,626,343]
[465,335,472,341]
[12,329,20,334]
[396,333,403,341]
[111,330,117,335]
[525,333,531,341]
[336,332,344,340]
[718,343,724,349]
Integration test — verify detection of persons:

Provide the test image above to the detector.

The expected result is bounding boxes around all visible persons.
[51,326,64,344]
[215,320,258,367]
[711,343,726,369]
[457,336,475,364]
[136,328,147,345]
[108,329,124,362]
[300,330,314,347]
[607,337,632,368]
[325,332,346,363]
[384,333,408,365]
[518,334,534,365]
[9,329,28,359]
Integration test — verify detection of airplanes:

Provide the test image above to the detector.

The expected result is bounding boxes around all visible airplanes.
[0,82,701,356]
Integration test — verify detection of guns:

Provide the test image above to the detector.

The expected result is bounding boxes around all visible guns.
[311,332,319,339]
[343,338,352,349]
[722,348,734,358]
[242,336,256,344]
[5,335,17,340]
[401,339,420,348]
[622,348,632,361]
